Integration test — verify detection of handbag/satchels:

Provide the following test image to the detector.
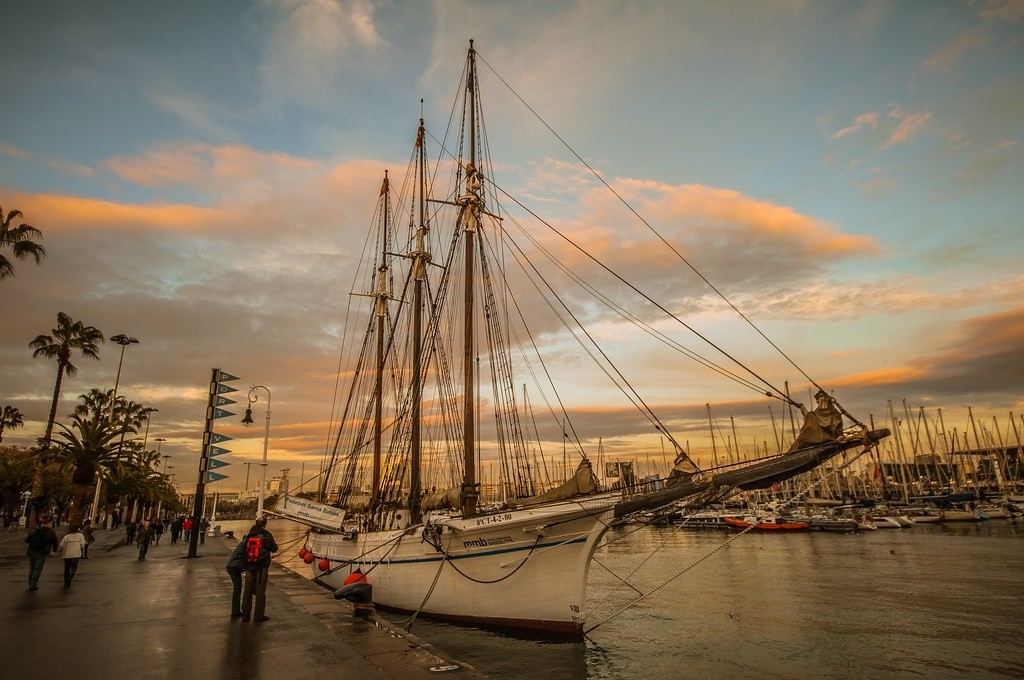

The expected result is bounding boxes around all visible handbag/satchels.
[88,536,96,543]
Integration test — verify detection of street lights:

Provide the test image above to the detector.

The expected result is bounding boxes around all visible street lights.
[148,439,180,520]
[89,334,139,526]
[142,407,159,460]
[240,386,272,521]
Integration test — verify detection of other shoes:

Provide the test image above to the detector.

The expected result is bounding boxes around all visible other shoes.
[257,616,268,622]
[29,587,39,590]
[242,619,249,621]
[143,556,145,558]
[84,556,88,559]
[80,557,83,559]
[231,612,243,618]
[138,557,141,559]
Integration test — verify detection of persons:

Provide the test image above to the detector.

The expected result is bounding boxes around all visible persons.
[126,518,136,544]
[81,520,96,559]
[199,518,210,544]
[226,525,256,619]
[241,516,278,621]
[26,521,58,590]
[110,506,120,530]
[95,513,102,524]
[59,524,86,588]
[13,508,58,527]
[137,518,168,551]
[135,520,154,560]
[3,516,11,527]
[170,515,193,544]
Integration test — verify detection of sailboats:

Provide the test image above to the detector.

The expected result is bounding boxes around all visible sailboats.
[262,38,890,632]
[429,398,1024,530]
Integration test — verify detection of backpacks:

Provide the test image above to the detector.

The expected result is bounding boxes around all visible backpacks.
[245,531,266,563]
